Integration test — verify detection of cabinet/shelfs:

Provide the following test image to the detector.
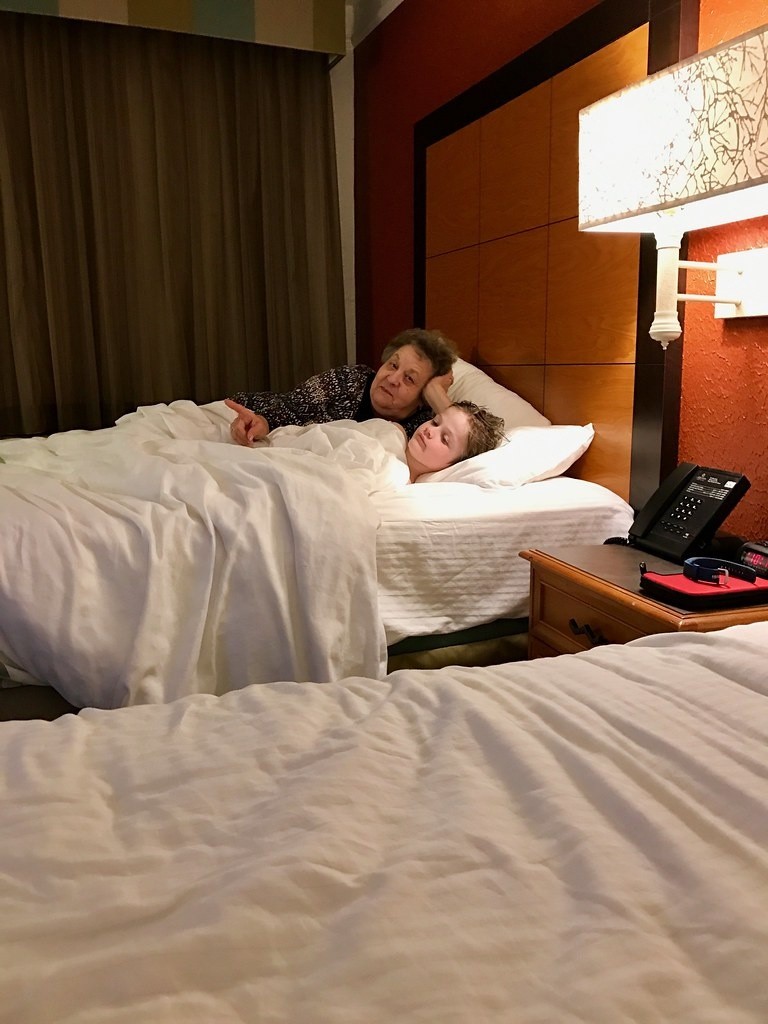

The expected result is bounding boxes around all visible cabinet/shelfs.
[518,544,768,660]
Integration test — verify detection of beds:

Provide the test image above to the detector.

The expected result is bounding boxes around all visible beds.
[0,401,636,713]
[0,621,768,1024]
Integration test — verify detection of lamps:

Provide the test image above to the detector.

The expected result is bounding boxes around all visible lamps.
[577,24,768,350]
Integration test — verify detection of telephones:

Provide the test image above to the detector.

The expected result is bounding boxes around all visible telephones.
[627,462,751,566]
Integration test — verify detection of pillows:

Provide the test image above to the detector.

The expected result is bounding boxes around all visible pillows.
[415,357,595,493]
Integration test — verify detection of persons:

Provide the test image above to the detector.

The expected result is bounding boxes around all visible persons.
[225,327,457,448]
[392,401,504,484]
